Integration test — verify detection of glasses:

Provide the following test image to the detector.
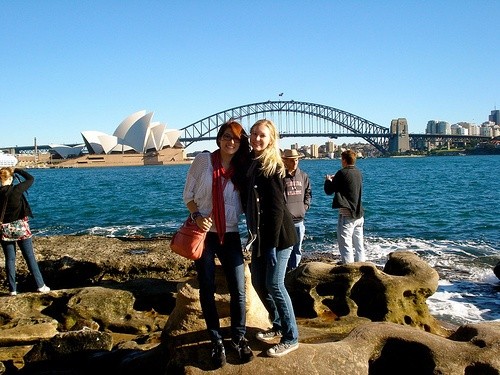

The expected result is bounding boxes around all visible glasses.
[221,133,242,143]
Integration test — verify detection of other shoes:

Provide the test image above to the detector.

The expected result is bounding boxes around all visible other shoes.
[36,284,50,292]
[209,338,226,368]
[230,338,254,361]
[9,291,16,295]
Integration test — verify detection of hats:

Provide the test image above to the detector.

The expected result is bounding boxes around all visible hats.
[281,149,302,158]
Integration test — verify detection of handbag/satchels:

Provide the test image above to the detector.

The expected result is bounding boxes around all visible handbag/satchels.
[170,216,207,260]
[0,216,32,241]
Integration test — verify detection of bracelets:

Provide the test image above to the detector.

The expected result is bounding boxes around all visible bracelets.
[190,212,203,220]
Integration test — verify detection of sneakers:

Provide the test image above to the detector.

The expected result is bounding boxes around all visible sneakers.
[256,327,283,340]
[266,342,299,356]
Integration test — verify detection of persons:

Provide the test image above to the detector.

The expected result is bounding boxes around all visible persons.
[183,122,253,368]
[0,167,50,296]
[324,150,366,264]
[280,149,312,267]
[240,119,300,357]
